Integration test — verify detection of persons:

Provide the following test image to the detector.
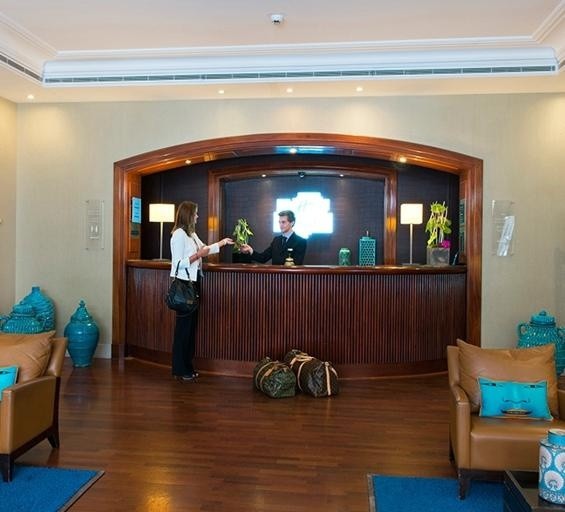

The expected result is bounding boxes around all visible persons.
[170,201,235,381]
[240,210,306,265]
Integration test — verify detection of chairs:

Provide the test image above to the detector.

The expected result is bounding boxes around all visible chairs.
[0,329,68,482]
[445,338,565,500]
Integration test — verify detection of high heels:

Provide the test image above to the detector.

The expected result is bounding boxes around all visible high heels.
[174,370,200,381]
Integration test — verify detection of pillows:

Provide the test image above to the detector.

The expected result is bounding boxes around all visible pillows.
[456,337,558,414]
[0,329,58,383]
[474,378,554,420]
[0,365,19,398]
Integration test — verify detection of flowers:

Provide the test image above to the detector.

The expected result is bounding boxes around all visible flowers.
[426,200,452,247]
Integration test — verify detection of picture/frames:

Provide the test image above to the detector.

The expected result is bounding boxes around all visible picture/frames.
[132,197,142,225]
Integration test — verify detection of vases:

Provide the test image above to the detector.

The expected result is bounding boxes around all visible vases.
[339,246,352,268]
[427,247,450,268]
[427,243,450,268]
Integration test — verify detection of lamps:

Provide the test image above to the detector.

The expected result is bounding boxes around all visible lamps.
[400,203,423,266]
[149,203,175,261]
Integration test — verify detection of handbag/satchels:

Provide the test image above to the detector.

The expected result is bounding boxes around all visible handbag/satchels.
[282,349,341,398]
[164,259,196,312]
[253,356,296,398]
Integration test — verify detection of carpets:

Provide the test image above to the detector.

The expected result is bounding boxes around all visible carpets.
[366,472,505,512]
[0,462,106,511]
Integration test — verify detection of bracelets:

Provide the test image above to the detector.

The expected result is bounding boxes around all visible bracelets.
[222,240,226,246]
[196,253,198,260]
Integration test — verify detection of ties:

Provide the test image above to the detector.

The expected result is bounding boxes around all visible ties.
[281,237,287,249]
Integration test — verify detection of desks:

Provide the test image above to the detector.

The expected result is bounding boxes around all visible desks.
[126,259,466,365]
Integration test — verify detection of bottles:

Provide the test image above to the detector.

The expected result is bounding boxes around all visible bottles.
[21,286,55,331]
[284,257,295,265]
[62,300,100,367]
[339,248,351,265]
[537,428,565,504]
[516,311,565,376]
[0,304,47,333]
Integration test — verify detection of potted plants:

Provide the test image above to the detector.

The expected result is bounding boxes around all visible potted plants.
[232,218,253,263]
